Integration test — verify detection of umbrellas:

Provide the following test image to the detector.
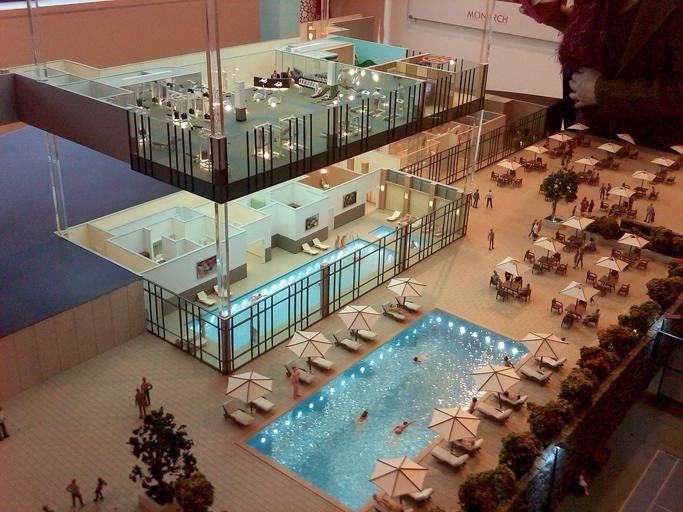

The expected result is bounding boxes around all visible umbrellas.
[566,122,590,131]
[336,304,382,341]
[519,332,569,369]
[386,276,427,305]
[368,455,429,503]
[225,369,273,404]
[427,406,481,450]
[470,363,521,408]
[285,329,332,373]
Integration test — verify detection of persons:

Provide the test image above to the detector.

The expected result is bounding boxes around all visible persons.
[503,355,515,369]
[389,304,408,315]
[66,478,86,507]
[135,389,147,419]
[484,190,494,209]
[310,85,323,97]
[321,174,329,189]
[290,366,302,399]
[373,494,405,512]
[334,235,341,249]
[248,293,267,301]
[472,189,480,208]
[93,477,107,502]
[371,89,381,111]
[340,235,346,248]
[418,57,432,68]
[354,410,369,440]
[413,353,426,363]
[287,67,292,78]
[43,504,54,512]
[458,439,476,445]
[141,378,153,406]
[388,420,416,443]
[468,397,477,414]
[503,390,520,400]
[271,70,277,79]
[519,0,683,147]
[490,132,682,326]
[0,407,10,441]
[395,210,431,254]
[488,228,495,250]
